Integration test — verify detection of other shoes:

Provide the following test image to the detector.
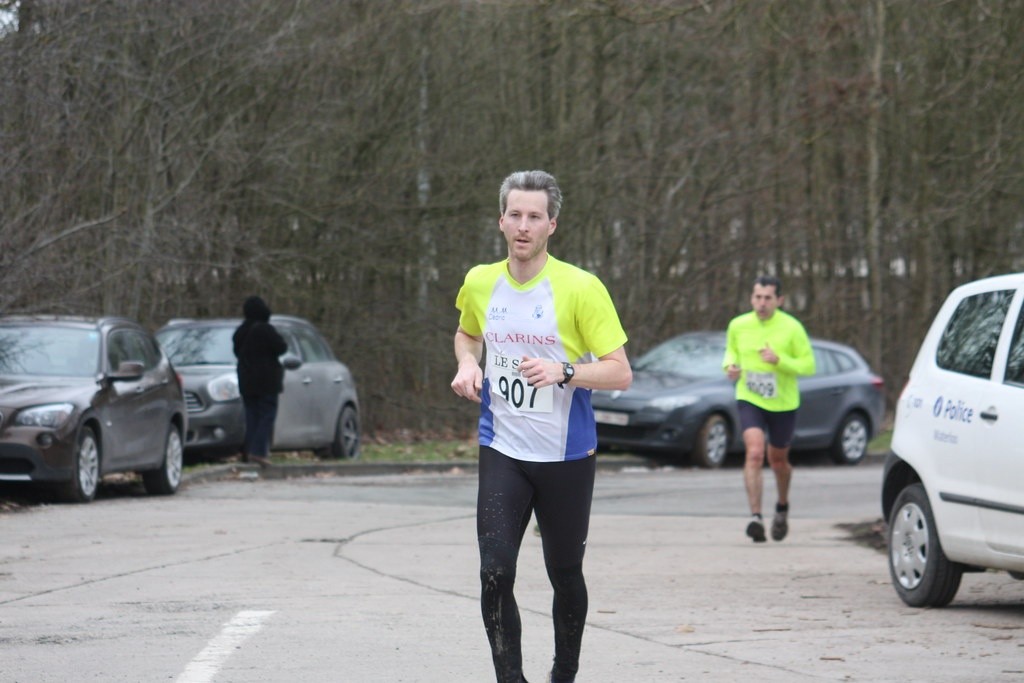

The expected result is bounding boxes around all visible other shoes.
[746,515,766,542]
[771,503,789,541]
[237,451,273,466]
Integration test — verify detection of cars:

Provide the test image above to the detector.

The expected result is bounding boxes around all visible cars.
[589,327,886,469]
[149,312,362,460]
[0,311,189,506]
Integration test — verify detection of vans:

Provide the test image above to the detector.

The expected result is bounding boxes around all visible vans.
[877,270,1024,609]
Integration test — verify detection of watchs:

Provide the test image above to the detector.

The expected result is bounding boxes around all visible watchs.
[557,362,574,389]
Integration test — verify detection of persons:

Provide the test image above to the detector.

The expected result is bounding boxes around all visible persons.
[722,276,816,543]
[232,295,288,466]
[450,170,633,683]
